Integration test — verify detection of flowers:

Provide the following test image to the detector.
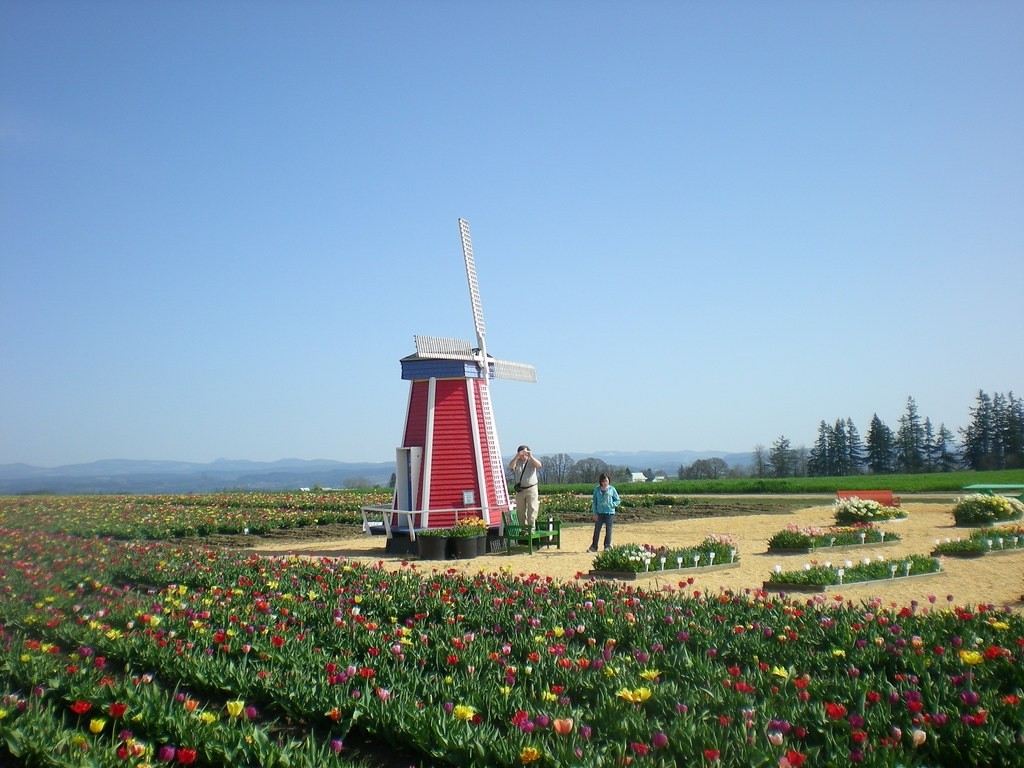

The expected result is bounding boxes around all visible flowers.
[457,516,490,535]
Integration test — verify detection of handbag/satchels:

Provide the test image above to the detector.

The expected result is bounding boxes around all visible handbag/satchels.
[514,483,523,492]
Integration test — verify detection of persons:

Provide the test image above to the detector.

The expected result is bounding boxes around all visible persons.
[586,473,621,553]
[509,445,542,535]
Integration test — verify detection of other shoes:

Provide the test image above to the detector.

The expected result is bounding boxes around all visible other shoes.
[604,546,612,551]
[519,529,534,536]
[587,546,597,552]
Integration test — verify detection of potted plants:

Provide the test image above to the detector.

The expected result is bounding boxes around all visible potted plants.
[418,525,480,560]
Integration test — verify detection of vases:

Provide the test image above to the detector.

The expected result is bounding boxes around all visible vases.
[476,535,486,556]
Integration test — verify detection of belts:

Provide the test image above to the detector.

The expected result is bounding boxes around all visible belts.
[522,483,538,489]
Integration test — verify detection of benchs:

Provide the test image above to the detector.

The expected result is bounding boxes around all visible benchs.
[838,490,901,509]
[501,509,562,554]
